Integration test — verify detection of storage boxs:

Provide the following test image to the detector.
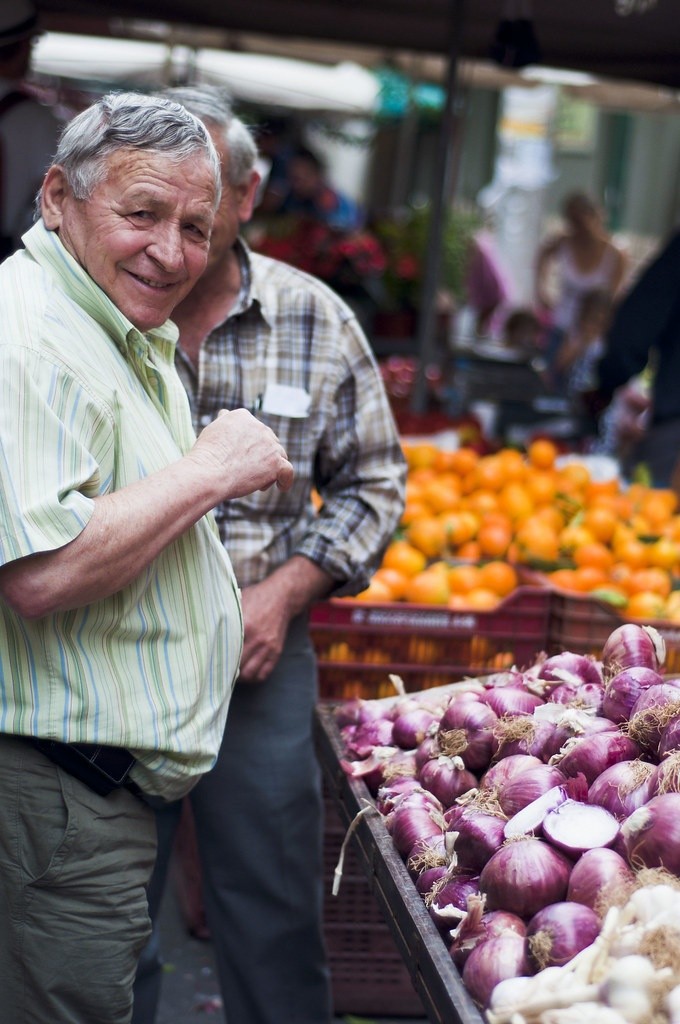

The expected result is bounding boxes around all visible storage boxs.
[309,567,680,1016]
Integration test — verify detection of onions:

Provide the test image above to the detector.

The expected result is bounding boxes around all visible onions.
[334,622,680,1004]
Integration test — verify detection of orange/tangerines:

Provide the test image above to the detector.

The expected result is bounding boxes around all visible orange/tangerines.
[308,439,680,701]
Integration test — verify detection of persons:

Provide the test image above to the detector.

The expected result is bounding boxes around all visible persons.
[2,74,501,360]
[0,88,296,1024]
[135,81,407,1024]
[506,189,627,396]
[571,237,680,495]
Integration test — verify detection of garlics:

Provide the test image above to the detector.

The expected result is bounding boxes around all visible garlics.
[481,883,680,1023]
